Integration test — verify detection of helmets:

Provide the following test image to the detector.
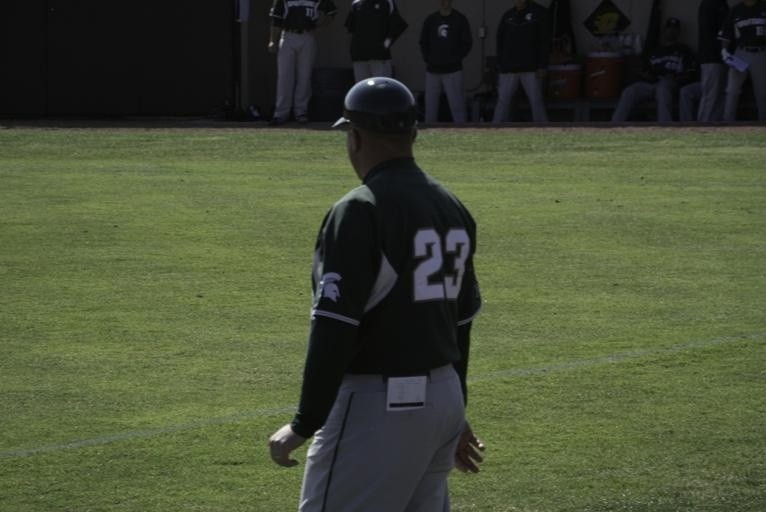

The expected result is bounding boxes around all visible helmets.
[332,77,419,134]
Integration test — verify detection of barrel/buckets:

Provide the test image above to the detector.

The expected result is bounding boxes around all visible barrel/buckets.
[544,62,584,97]
[582,49,625,98]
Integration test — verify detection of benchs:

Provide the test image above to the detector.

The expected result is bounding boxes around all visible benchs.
[469,93,759,123]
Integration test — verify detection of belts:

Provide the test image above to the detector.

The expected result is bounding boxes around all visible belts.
[740,45,765,52]
[285,28,310,33]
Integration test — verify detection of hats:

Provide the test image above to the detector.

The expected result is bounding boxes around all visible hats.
[667,18,680,30]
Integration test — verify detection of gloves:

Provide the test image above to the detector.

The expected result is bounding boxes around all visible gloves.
[721,48,730,61]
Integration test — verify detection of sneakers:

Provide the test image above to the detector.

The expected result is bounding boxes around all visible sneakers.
[269,119,288,126]
[297,116,308,124]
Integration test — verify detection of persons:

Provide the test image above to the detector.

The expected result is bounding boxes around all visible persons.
[267,0,337,126]
[610,0,765,125]
[418,1,474,126]
[270,77,486,512]
[331,0,408,126]
[490,1,553,123]
